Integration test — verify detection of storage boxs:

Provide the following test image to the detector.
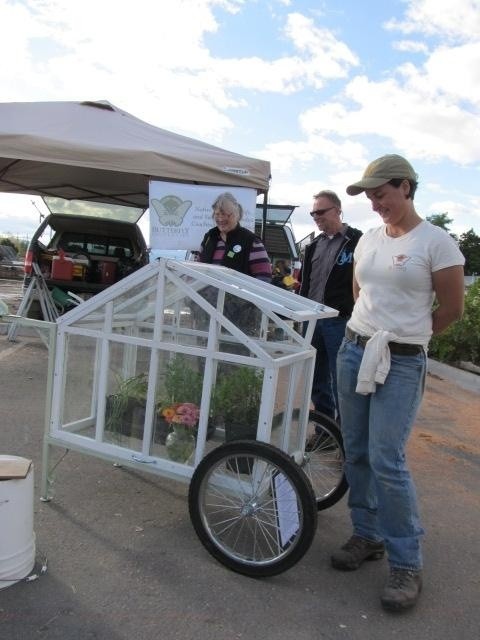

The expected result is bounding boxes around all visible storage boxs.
[51,255,73,281]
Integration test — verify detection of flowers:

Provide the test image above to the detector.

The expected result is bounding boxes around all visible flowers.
[272,267,300,291]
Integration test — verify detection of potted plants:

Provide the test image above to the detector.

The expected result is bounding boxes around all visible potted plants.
[104,359,266,475]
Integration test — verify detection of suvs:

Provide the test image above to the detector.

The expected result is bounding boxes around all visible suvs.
[20,194,151,312]
[183,224,300,307]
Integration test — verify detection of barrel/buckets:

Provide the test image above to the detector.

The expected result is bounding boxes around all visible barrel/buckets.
[50,249,73,281]
[95,262,116,284]
[0,454,48,590]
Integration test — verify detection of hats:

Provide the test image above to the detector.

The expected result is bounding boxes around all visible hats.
[346,153,418,196]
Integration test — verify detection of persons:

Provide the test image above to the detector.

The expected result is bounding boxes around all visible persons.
[293,189,364,454]
[182,191,274,384]
[326,152,467,614]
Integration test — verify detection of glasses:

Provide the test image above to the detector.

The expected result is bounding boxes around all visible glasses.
[309,207,337,217]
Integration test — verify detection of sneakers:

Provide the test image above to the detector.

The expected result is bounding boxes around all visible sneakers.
[330,532,386,572]
[378,566,424,610]
[302,433,337,451]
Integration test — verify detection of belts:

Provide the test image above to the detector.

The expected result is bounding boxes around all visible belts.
[345,325,428,358]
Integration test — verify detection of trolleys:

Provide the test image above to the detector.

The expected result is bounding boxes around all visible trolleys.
[40,255,349,575]
